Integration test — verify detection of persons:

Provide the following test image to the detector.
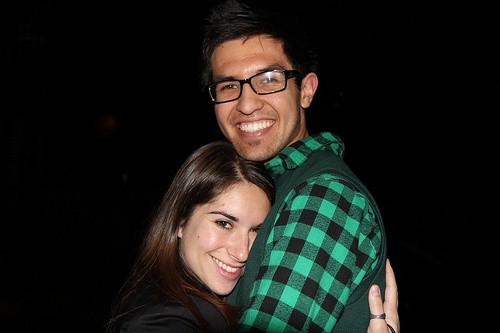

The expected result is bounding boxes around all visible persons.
[107,141,401,333]
[203,1,388,333]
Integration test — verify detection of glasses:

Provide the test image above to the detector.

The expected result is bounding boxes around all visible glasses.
[207,68,300,104]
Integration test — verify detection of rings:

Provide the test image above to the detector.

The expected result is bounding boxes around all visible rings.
[369,313,385,319]
